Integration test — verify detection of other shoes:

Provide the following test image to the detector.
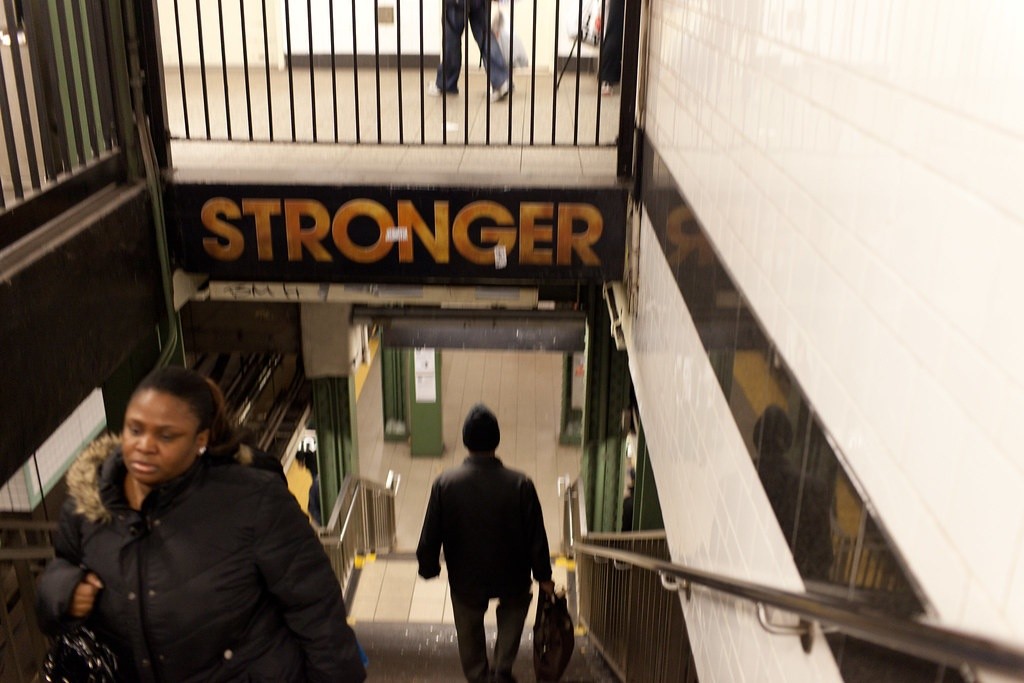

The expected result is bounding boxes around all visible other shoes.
[428,82,457,97]
[492,82,508,102]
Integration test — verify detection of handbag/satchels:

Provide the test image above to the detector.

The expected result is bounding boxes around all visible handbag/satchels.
[533,587,574,683]
[40,628,117,683]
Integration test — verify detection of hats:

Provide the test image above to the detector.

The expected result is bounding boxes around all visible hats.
[462,404,500,452]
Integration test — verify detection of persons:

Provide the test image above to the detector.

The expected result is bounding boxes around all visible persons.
[596,0,625,95]
[417,404,555,683]
[751,405,835,580]
[426,0,515,101]
[29,368,369,683]
